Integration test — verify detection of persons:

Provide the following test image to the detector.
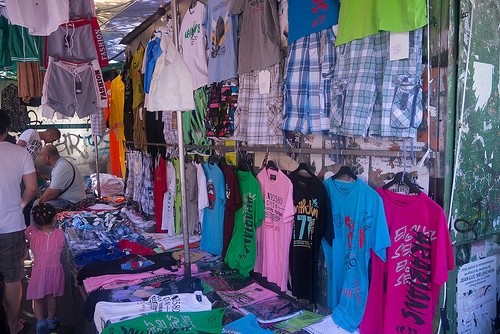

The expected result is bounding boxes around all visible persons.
[23,203,66,334]
[0,109,87,334]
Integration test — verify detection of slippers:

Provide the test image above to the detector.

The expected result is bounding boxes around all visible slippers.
[17,316,32,334]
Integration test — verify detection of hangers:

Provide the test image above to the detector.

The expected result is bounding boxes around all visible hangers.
[126,140,422,196]
[125,0,197,63]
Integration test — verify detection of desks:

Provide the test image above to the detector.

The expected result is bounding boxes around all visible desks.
[53,201,356,334]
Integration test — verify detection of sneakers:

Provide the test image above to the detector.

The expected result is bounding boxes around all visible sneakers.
[36,320,50,334]
[47,317,58,330]
